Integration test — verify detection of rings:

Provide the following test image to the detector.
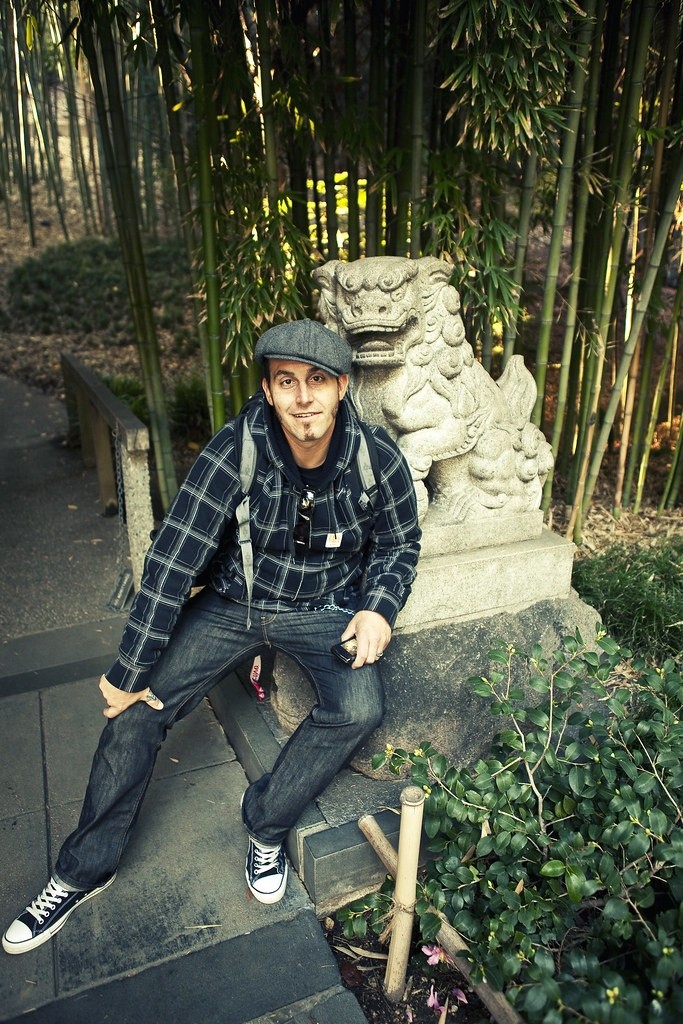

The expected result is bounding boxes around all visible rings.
[376,652,384,657]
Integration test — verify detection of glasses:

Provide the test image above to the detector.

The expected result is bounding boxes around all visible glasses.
[292,485,318,551]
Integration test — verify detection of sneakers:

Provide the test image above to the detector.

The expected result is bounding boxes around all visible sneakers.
[240,792,289,905]
[1,870,118,955]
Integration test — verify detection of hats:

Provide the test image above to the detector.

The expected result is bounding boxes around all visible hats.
[254,317,353,377]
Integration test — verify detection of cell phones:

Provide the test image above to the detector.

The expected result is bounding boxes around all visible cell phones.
[331,636,357,665]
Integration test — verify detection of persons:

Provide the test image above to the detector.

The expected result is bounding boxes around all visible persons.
[1,318,421,954]
